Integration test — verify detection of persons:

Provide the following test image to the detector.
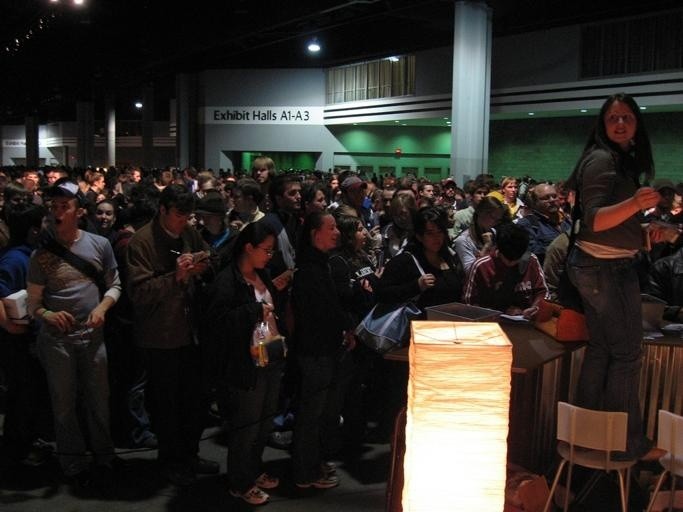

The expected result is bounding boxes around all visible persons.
[567,93,667,462]
[2,156,682,503]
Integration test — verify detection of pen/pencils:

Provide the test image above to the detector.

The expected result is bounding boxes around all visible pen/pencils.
[170,249,183,255]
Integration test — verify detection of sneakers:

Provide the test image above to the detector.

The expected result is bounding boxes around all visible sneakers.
[229,472,280,504]
[296,463,340,489]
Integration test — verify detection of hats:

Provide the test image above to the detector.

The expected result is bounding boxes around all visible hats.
[342,177,368,191]
[193,193,227,217]
[43,182,86,203]
[655,179,675,192]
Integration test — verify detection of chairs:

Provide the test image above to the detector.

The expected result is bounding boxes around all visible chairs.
[646,408,683,512]
[541,402,638,510]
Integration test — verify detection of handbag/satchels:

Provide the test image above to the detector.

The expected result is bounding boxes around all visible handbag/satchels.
[352,301,422,354]
[555,267,586,316]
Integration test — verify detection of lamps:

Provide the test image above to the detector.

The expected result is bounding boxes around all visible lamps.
[401,321,512,511]
